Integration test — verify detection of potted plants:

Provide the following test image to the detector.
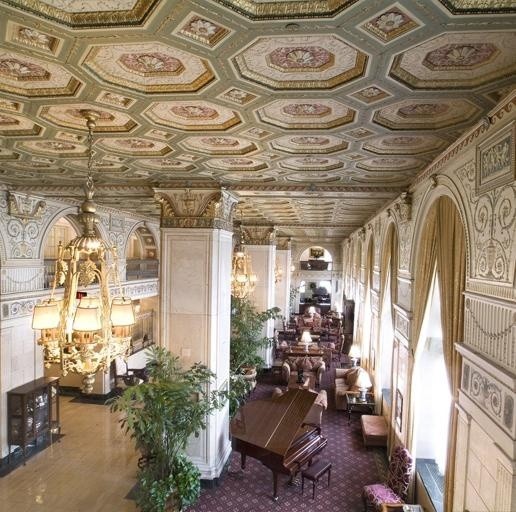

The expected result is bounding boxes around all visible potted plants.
[230,294,286,391]
[104,346,248,512]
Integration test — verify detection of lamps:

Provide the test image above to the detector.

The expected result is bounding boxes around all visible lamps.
[356,372,372,402]
[348,345,360,366]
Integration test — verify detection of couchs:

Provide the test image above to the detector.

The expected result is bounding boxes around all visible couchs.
[335,366,374,412]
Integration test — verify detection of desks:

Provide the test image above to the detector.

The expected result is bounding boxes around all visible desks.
[381,504,424,512]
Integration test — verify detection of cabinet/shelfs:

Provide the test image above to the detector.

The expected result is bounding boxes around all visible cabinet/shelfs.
[7,377,61,465]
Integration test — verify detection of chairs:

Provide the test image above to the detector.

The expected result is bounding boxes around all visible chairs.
[362,444,416,512]
[273,308,344,362]
[272,356,326,425]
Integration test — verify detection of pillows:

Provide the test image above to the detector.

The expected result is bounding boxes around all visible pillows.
[345,366,371,391]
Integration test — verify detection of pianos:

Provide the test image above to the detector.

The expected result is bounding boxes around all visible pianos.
[229,388,327,501]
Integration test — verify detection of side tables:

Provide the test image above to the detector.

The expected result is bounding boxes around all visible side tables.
[341,363,360,369]
[346,392,376,425]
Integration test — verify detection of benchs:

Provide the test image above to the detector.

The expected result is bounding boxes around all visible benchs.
[302,457,331,498]
[360,414,390,451]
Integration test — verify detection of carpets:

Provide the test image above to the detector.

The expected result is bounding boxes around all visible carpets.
[69,388,122,405]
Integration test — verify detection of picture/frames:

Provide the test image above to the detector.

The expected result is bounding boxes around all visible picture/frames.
[395,388,403,432]
[476,113,516,196]
